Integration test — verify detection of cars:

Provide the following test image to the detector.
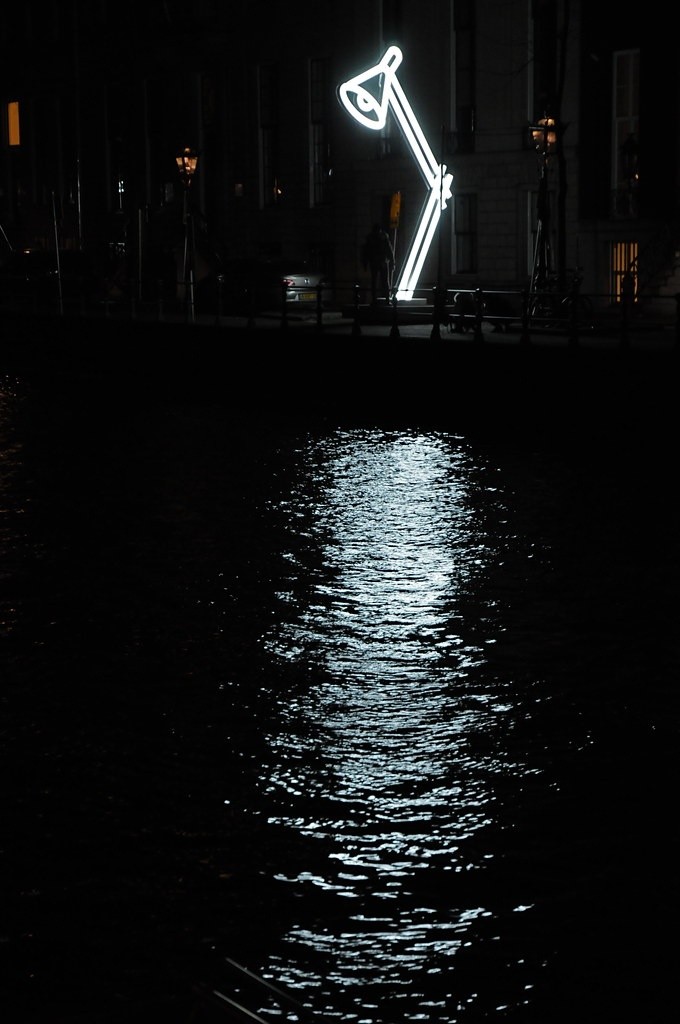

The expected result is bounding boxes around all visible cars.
[202,256,335,311]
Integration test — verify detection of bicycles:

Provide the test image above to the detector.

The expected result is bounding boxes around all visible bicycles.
[523,267,594,333]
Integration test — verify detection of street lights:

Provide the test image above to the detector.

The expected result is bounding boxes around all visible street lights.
[524,112,561,292]
[175,143,199,317]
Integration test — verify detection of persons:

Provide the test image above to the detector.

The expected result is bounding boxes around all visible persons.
[361,224,394,304]
[449,293,477,334]
